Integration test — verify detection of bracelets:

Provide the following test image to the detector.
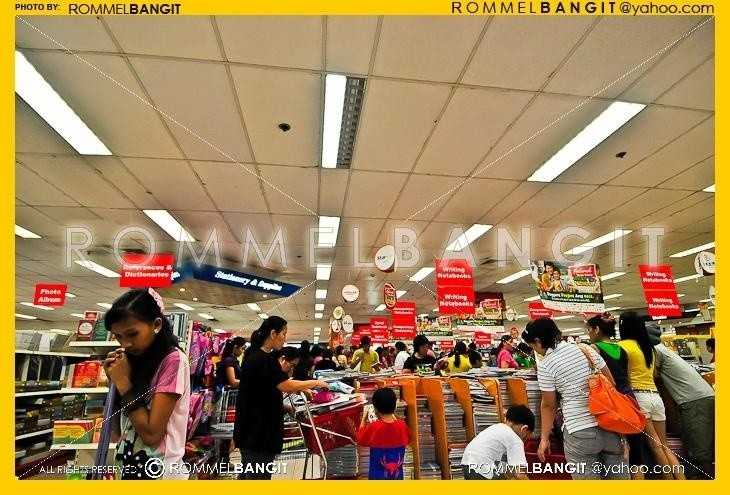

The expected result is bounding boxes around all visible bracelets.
[119,386,146,417]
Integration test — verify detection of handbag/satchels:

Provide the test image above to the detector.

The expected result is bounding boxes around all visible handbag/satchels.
[578,341,645,435]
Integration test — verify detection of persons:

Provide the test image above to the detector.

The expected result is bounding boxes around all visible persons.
[513,343,533,368]
[103,288,191,480]
[424,345,434,358]
[390,348,396,365]
[394,342,410,371]
[546,269,565,293]
[401,335,436,374]
[496,335,519,369]
[429,342,434,350]
[459,404,536,479]
[521,318,631,480]
[341,349,350,358]
[469,343,479,355]
[337,345,344,355]
[585,310,646,480]
[348,346,357,355]
[487,347,497,367]
[350,335,383,374]
[314,351,337,372]
[310,345,321,364]
[575,336,581,343]
[652,345,715,480]
[214,336,247,471]
[540,262,554,291]
[234,317,329,481]
[355,388,413,479]
[270,346,300,375]
[616,307,687,480]
[378,348,388,368]
[469,351,483,368]
[437,351,446,360]
[707,339,715,364]
[293,339,316,382]
[447,341,473,375]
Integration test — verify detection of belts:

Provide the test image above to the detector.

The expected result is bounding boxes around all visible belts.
[633,389,660,394]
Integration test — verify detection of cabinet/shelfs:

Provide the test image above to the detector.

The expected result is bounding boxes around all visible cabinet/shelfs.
[353,377,502,480]
[15,350,96,479]
[51,338,186,449]
[481,379,534,438]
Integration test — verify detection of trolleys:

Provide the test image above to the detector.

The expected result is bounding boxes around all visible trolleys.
[285,389,368,481]
[209,384,242,480]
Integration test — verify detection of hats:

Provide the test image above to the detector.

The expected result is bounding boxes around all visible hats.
[646,322,662,344]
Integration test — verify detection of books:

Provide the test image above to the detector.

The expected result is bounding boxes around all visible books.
[357,446,372,478]
[513,370,541,437]
[212,423,236,441]
[388,386,401,400]
[359,388,375,402]
[394,401,408,419]
[326,447,357,477]
[403,449,414,480]
[498,378,510,418]
[416,395,442,480]
[467,379,501,437]
[441,381,468,479]
[665,431,683,458]
